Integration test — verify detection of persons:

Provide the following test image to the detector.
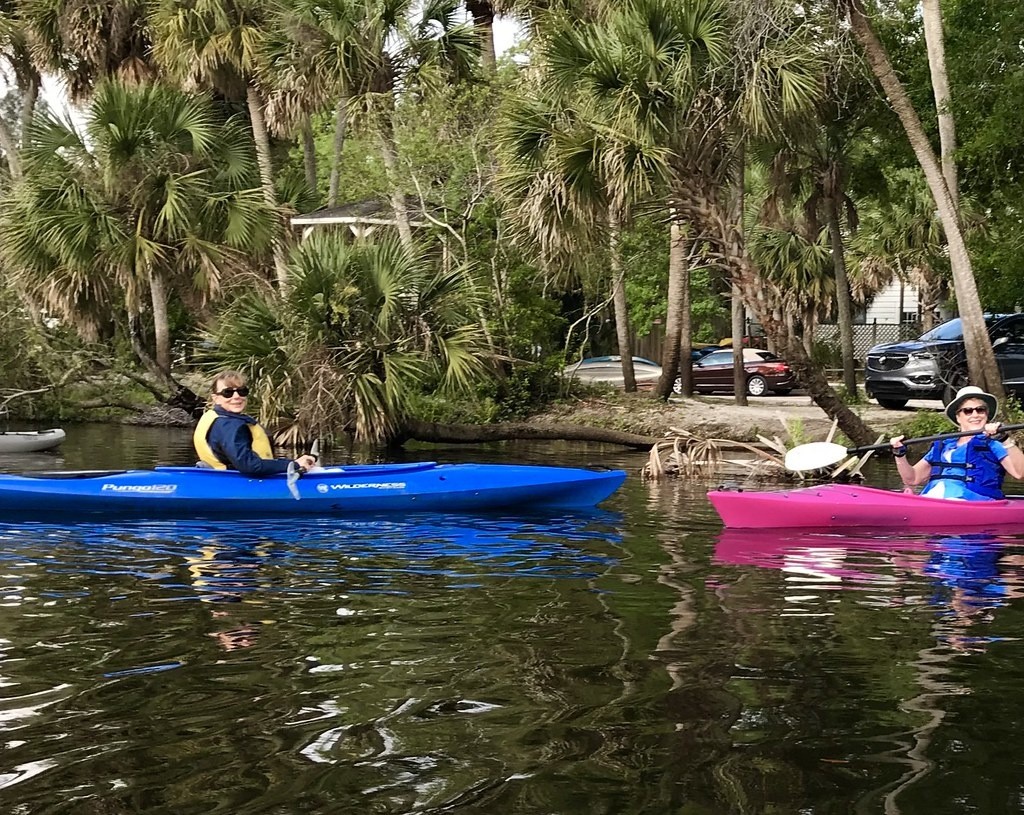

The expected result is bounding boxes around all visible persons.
[192,369,316,478]
[889,385,1024,502]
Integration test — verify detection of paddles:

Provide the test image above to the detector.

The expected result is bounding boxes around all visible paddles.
[781,421,1024,473]
[282,437,322,505]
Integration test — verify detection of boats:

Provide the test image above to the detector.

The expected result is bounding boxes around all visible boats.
[1,427,67,453]
[705,482,1024,531]
[0,460,629,521]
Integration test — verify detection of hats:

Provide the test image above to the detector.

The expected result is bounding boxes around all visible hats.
[945,385,998,427]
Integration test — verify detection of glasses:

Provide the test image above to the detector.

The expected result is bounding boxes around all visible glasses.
[955,406,987,414]
[213,386,249,398]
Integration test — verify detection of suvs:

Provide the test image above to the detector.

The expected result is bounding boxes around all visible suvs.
[864,313,1024,412]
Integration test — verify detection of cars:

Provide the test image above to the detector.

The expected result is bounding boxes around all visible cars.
[672,347,796,398]
